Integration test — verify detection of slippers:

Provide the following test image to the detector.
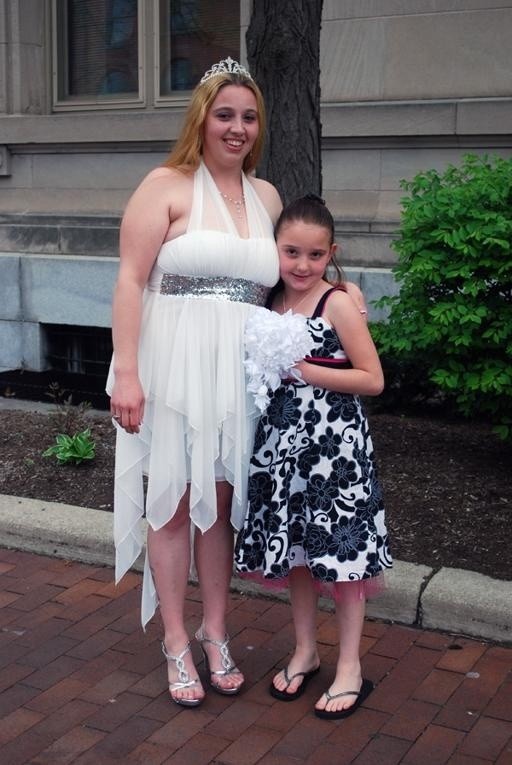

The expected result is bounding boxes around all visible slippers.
[313,678,375,720]
[270,663,321,701]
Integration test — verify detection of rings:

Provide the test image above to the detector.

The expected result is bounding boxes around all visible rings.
[106,57,367,708]
[113,416,120,419]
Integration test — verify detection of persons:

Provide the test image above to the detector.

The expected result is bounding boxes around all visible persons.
[233,195,392,720]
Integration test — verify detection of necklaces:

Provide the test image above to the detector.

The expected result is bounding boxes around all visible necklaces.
[282,281,321,314]
[209,167,245,213]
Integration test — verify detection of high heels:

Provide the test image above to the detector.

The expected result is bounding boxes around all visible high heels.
[160,639,205,707]
[194,622,245,696]
